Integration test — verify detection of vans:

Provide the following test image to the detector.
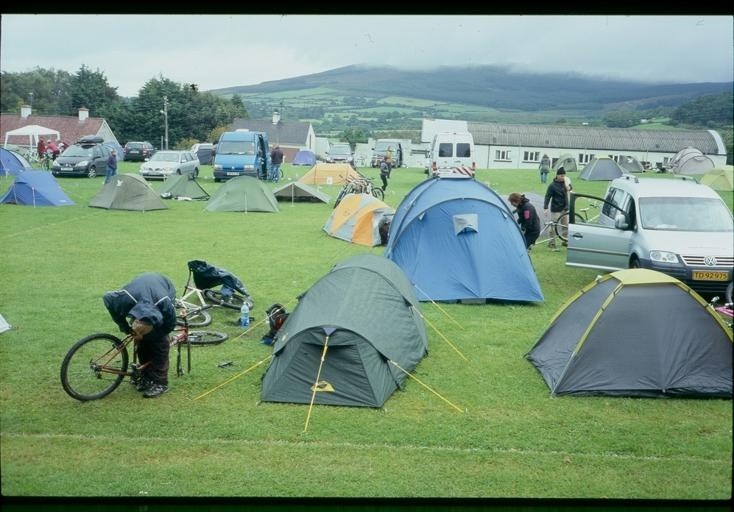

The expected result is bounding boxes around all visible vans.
[211,128,271,184]
[370,139,403,168]
[138,149,201,180]
[428,130,477,180]
[563,175,734,298]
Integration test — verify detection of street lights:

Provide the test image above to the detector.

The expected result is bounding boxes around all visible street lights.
[157,94,170,152]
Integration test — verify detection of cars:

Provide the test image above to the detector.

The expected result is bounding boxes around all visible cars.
[51,135,116,178]
[190,142,213,151]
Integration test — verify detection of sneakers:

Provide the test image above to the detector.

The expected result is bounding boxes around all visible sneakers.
[144,383,167,398]
[136,378,152,391]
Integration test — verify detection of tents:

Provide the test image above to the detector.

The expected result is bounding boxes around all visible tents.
[88,173,210,211]
[299,163,362,186]
[322,193,397,248]
[201,176,278,213]
[523,269,734,397]
[274,181,329,203]
[4,125,60,151]
[260,253,429,410]
[2,148,73,207]
[378,176,545,305]
[552,144,733,194]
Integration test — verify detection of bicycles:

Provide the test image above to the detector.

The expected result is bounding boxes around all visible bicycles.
[726,280,734,303]
[707,296,734,328]
[528,212,587,253]
[580,201,603,224]
[333,174,385,205]
[171,259,253,327]
[270,163,283,183]
[22,147,44,165]
[40,152,53,171]
[60,310,230,403]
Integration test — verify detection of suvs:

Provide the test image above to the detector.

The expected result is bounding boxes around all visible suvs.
[123,141,154,161]
[324,141,355,168]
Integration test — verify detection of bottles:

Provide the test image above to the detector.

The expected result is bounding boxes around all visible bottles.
[240,301,250,327]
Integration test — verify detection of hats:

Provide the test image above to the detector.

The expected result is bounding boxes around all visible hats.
[557,167,565,175]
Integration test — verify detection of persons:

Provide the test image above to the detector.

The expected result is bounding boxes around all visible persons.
[538,154,550,183]
[507,193,540,249]
[268,144,283,183]
[380,156,390,191]
[101,271,176,397]
[38,139,60,160]
[543,168,573,250]
[385,146,396,178]
[104,151,117,183]
